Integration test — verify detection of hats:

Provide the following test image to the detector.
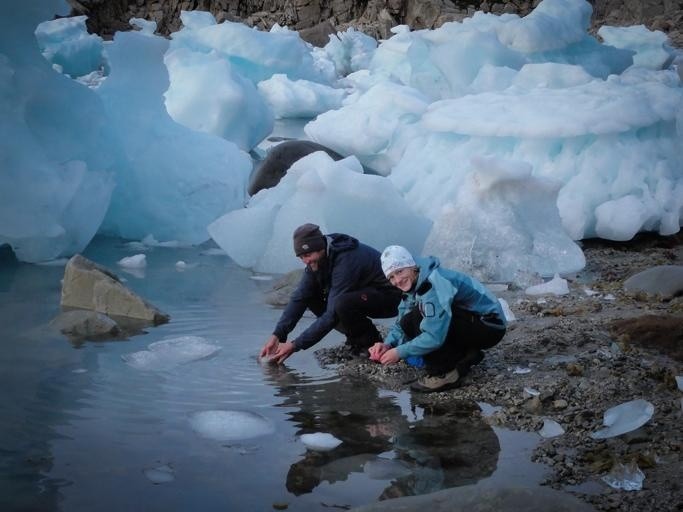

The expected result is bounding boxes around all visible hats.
[292,222,327,257]
[380,244,417,280]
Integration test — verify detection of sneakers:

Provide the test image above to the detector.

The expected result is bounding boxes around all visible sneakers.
[410,367,462,394]
[462,348,485,369]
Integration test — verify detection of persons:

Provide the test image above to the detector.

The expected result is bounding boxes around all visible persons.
[363,396,500,502]
[365,244,508,395]
[258,223,404,366]
[265,365,403,497]
